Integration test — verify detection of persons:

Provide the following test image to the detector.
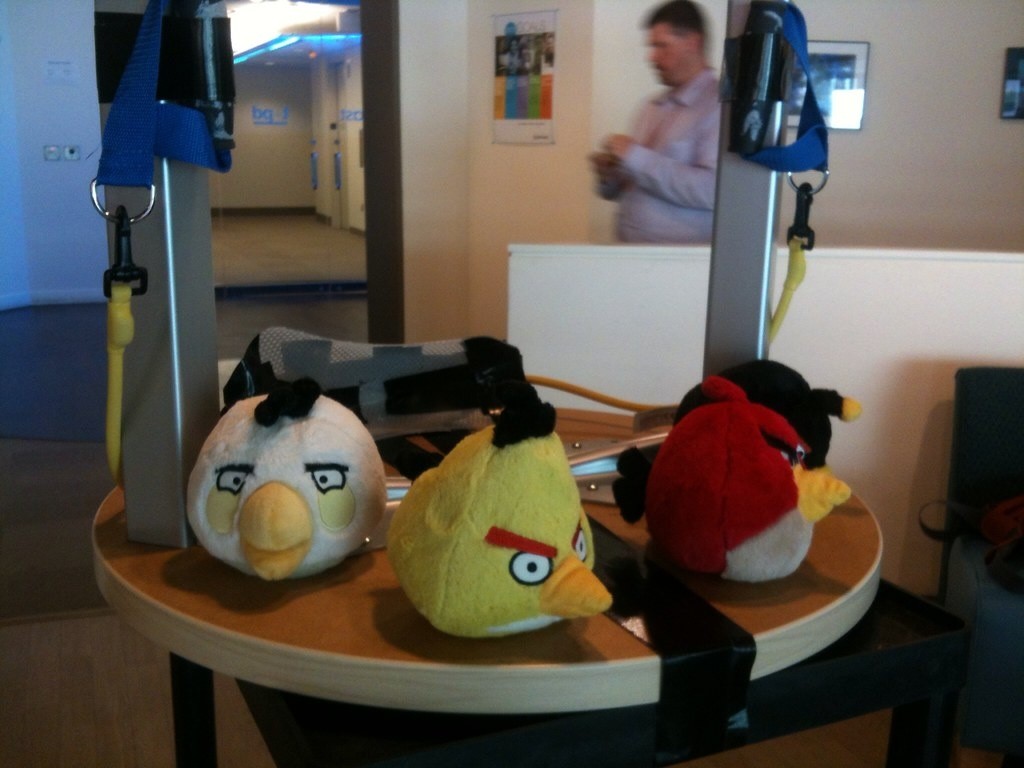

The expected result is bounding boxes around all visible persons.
[588,2,721,245]
[496,31,555,75]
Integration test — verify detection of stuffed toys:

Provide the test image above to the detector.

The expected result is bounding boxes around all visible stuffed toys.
[390,388,612,639]
[614,359,862,584]
[186,375,387,582]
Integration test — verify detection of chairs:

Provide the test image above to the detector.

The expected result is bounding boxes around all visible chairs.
[938,368,1023,755]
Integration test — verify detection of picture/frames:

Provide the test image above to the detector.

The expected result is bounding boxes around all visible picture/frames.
[808,40,869,130]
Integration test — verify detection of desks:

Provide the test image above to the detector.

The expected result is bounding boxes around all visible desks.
[165,578,976,767]
